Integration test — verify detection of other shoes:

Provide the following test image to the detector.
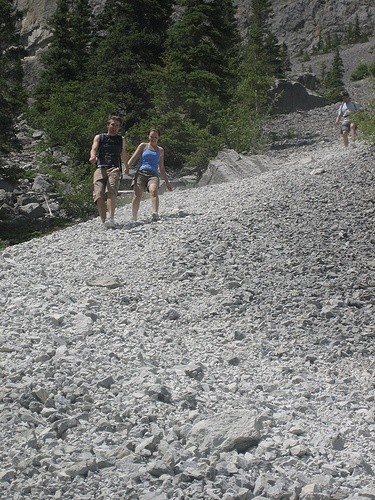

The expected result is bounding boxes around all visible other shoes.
[152,212,159,220]
[130,218,136,227]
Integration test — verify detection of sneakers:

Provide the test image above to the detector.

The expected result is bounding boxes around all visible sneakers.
[101,219,114,229]
[342,142,355,151]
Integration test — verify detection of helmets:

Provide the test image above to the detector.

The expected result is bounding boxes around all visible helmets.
[340,91,348,97]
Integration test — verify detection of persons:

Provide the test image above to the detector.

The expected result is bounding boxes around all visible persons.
[89,115,129,230]
[335,91,363,150]
[125,128,173,227]
